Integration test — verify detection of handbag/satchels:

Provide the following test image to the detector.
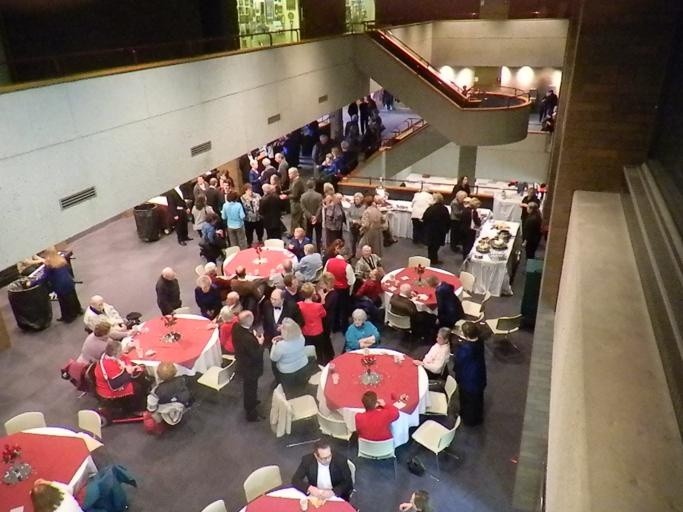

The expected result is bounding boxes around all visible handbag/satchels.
[407,460,426,477]
[199,239,221,258]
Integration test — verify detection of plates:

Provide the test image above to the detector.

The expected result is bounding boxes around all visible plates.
[476,240,508,254]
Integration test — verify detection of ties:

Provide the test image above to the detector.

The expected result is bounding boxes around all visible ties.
[368,258,372,269]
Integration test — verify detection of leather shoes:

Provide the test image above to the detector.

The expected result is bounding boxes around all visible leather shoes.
[179,237,193,245]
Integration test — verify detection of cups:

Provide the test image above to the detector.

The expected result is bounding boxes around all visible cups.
[390,392,397,401]
[284,250,288,256]
[332,373,339,384]
[300,499,308,512]
[330,364,336,374]
[396,358,401,366]
[254,269,260,276]
[136,348,143,358]
[363,348,369,356]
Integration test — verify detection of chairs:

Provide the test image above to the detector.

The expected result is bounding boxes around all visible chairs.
[5,412,46,435]
[243,465,281,504]
[345,459,357,500]
[63,307,238,410]
[192,239,322,295]
[268,345,463,482]
[201,499,227,512]
[373,255,521,355]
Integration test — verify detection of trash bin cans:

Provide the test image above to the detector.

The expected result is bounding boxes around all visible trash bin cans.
[7,278,52,332]
[520,259,544,319]
[133,203,162,243]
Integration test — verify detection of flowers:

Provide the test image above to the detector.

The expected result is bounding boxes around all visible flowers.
[1,442,21,462]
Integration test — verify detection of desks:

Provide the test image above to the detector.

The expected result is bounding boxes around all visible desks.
[312,347,429,448]
[404,173,534,223]
[221,246,299,284]
[379,266,463,309]
[458,219,521,298]
[340,194,492,245]
[120,312,222,378]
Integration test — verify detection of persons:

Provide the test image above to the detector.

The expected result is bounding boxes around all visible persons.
[462,85,471,96]
[539,90,558,131]
[82,267,192,413]
[399,490,431,512]
[293,179,384,364]
[454,321,487,426]
[16,250,84,325]
[306,120,359,181]
[410,176,482,265]
[192,134,310,263]
[291,438,352,501]
[345,95,381,157]
[414,327,451,379]
[383,89,395,110]
[167,185,193,246]
[194,262,311,422]
[30,479,84,512]
[355,390,399,441]
[520,187,543,257]
[389,276,464,336]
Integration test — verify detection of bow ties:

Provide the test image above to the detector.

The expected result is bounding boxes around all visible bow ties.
[275,307,280,310]
